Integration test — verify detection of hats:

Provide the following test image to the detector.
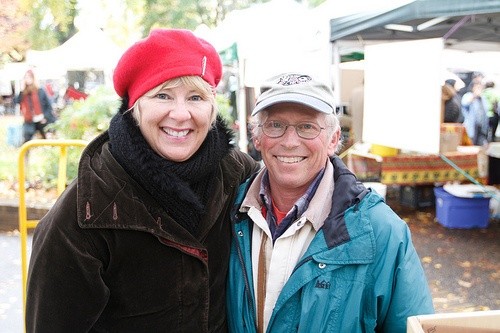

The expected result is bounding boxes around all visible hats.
[250,71,337,117]
[114,28,219,108]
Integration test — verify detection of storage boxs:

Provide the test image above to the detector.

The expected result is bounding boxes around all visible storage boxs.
[433,187,490,229]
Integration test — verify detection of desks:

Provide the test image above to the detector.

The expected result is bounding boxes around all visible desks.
[347,148,481,211]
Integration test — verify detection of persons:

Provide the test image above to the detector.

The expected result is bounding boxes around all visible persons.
[227,70,434,333]
[43,77,59,110]
[443,74,500,186]
[6,70,53,145]
[25,26,270,333]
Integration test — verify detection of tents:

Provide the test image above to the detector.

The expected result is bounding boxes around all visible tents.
[0,0,500,149]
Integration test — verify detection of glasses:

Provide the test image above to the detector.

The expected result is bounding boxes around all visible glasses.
[255,119,333,138]
